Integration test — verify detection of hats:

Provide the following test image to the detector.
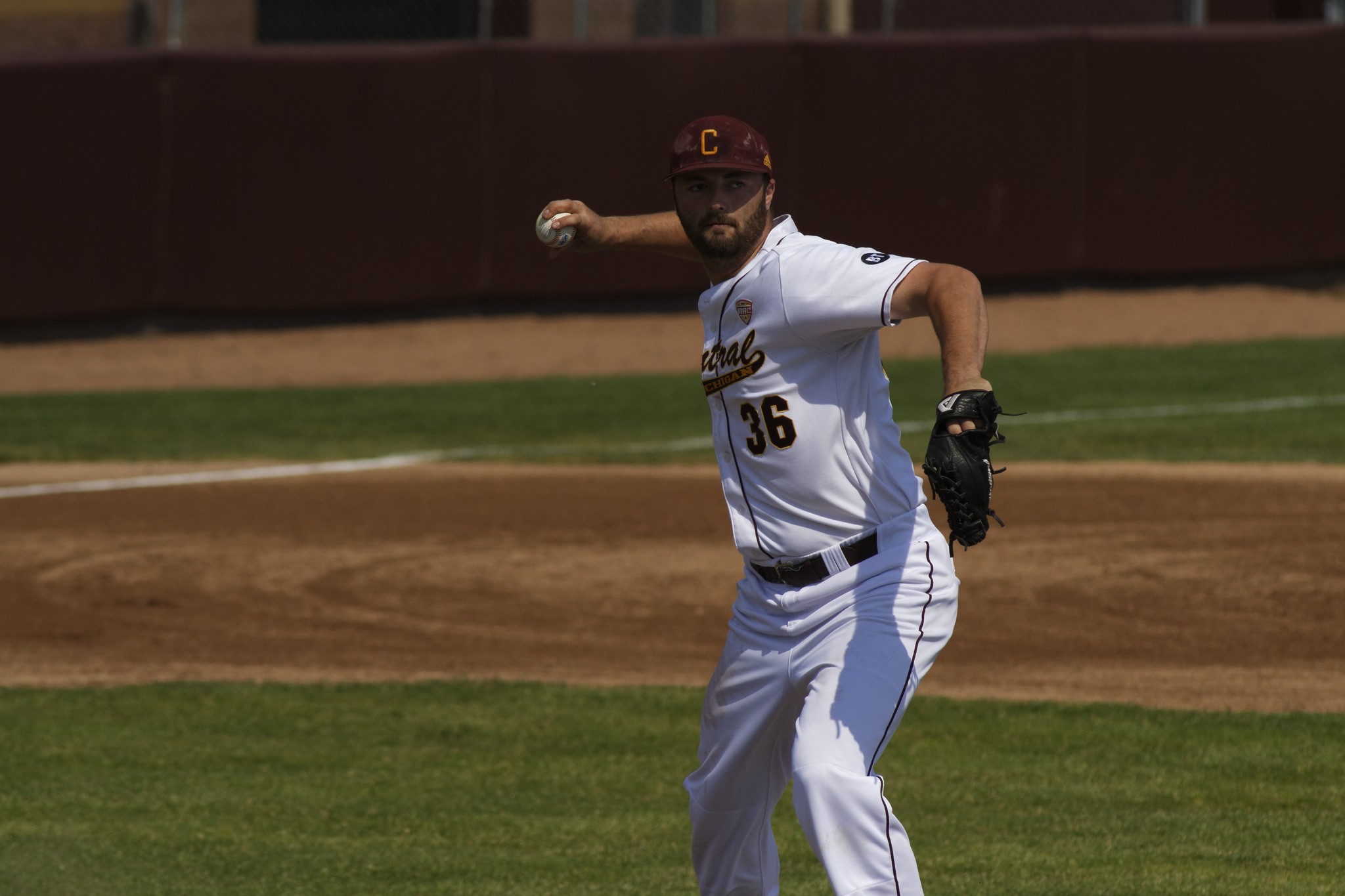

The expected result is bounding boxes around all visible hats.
[664,119,771,179]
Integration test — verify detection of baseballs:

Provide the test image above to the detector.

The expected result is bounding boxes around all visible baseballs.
[535,211,576,248]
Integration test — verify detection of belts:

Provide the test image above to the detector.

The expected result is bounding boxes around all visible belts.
[749,534,879,586]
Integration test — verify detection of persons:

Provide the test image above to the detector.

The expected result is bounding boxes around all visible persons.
[543,118,998,896]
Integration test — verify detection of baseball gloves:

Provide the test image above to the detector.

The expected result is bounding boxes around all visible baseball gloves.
[925,390,1001,546]
